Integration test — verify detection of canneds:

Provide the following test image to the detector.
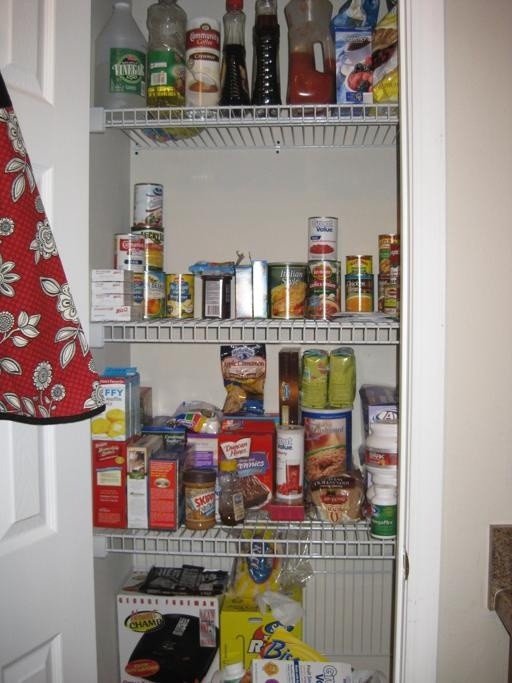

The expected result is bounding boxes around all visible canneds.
[269,216,341,319]
[181,467,217,530]
[114,184,195,319]
[343,254,373,312]
[185,16,221,118]
[379,234,400,314]
[200,274,231,318]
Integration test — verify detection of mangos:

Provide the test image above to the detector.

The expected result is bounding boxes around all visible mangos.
[91,409,126,438]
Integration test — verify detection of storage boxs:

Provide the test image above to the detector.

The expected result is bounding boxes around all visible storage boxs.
[89,343,399,540]
[216,579,306,680]
[248,654,356,681]
[115,566,232,681]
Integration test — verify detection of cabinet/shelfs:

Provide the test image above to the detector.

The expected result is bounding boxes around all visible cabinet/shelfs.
[88,95,403,565]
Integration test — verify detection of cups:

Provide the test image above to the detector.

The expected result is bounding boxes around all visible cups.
[286,456,300,492]
[338,37,372,66]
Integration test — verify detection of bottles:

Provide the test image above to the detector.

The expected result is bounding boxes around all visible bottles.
[94,0,335,120]
[362,423,397,538]
[224,662,244,682]
[219,459,246,524]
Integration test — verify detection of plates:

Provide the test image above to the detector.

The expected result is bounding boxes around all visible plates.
[252,477,272,510]
[340,60,366,93]
